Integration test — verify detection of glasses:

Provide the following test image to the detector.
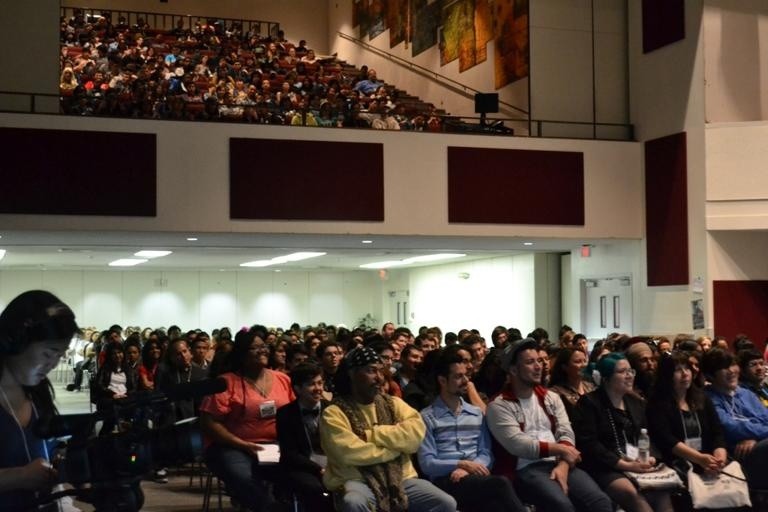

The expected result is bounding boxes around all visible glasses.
[615,368,637,377]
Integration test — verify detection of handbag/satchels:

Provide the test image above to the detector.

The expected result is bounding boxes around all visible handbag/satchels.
[620,453,686,490]
[687,461,752,509]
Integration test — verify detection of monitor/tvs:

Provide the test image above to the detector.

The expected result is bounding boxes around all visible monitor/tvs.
[475,93,499,113]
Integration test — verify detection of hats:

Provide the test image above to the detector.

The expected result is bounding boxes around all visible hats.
[500,337,537,368]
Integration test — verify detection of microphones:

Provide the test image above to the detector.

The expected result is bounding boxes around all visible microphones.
[113,376,229,416]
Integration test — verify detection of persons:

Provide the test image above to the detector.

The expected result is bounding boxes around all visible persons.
[61,13,446,132]
[64,321,767,512]
[0,288,83,512]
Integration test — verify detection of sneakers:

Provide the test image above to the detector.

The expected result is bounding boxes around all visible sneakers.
[150,467,169,483]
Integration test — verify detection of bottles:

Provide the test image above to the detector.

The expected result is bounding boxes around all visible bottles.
[638,428,651,466]
